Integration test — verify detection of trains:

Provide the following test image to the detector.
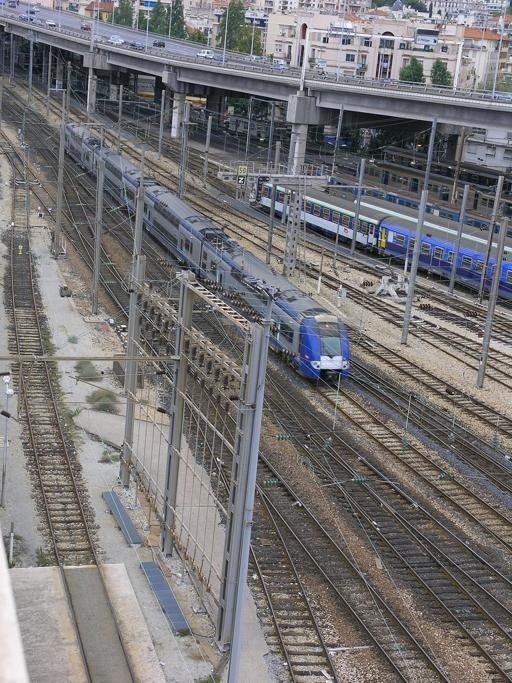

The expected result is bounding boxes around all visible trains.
[248,143,512,306]
[58,119,353,383]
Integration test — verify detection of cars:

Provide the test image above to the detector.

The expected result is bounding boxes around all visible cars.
[108,34,125,45]
[480,90,511,102]
[153,39,165,47]
[244,54,261,66]
[128,39,145,50]
[80,22,91,31]
[312,69,333,81]
[0,0,41,23]
[212,53,227,62]
[46,18,56,26]
[377,76,401,89]
[269,63,291,72]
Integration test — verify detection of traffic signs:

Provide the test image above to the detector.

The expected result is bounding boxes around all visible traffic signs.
[238,166,247,175]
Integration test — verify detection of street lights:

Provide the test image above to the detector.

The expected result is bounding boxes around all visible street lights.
[144,14,150,52]
[112,5,119,23]
[205,25,212,47]
[1,375,14,506]
[492,30,507,95]
[249,13,256,56]
[336,10,345,82]
[222,6,229,63]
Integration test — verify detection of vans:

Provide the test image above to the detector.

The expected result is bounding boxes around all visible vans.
[196,48,215,60]
